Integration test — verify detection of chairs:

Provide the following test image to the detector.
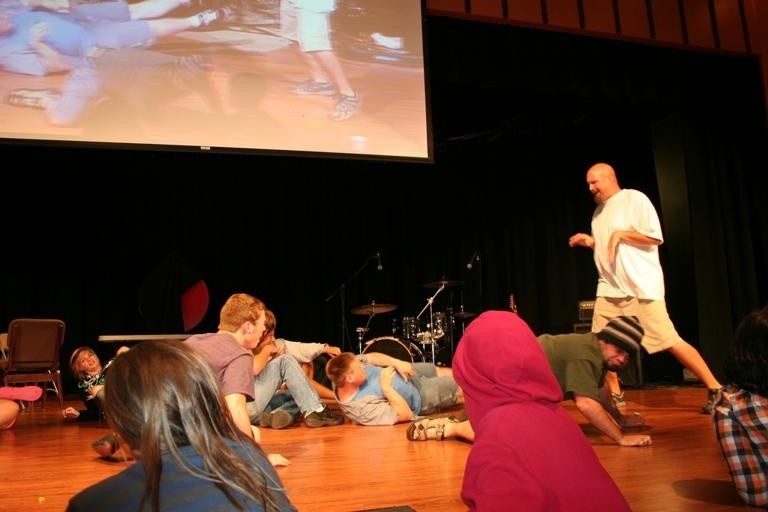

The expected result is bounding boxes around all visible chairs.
[0,319,66,410]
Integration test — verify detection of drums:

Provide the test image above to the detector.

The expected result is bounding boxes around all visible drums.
[416,330,436,344]
[361,336,425,364]
[426,312,448,337]
[393,316,420,339]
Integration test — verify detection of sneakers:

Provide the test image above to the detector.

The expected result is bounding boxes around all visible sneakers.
[258,412,295,429]
[305,403,344,427]
[91,433,120,457]
[703,384,725,414]
[287,78,338,97]
[611,390,626,408]
[7,89,59,109]
[199,6,233,27]
[328,90,361,120]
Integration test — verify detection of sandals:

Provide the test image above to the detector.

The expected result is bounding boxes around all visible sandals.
[407,416,460,440]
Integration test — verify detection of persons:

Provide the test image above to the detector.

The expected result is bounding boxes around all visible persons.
[92,292,292,466]
[65,338,299,511]
[62,343,132,425]
[711,304,768,508]
[569,163,725,412]
[324,351,465,426]
[0,380,42,432]
[242,308,344,430]
[405,316,653,447]
[0,1,367,135]
[453,309,631,511]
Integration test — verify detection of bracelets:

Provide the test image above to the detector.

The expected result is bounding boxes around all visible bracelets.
[608,390,624,410]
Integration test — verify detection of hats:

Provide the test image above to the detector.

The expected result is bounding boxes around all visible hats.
[596,316,644,358]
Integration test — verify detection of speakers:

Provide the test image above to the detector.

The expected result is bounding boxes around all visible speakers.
[570,319,685,387]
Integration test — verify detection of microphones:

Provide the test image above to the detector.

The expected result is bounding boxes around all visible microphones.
[377,252,383,270]
[467,247,478,269]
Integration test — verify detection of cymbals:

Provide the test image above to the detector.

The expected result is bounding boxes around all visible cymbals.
[353,303,397,314]
[424,281,460,288]
[454,312,474,318]
